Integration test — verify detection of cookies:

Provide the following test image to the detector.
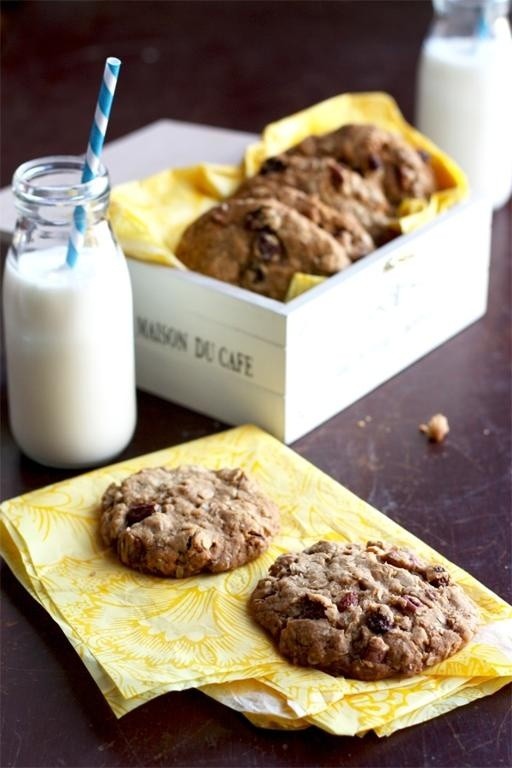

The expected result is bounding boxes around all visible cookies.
[178,121,433,305]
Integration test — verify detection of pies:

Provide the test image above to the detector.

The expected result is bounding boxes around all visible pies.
[98,464,276,580]
[249,541,479,679]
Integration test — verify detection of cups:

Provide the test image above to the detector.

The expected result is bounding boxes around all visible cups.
[414,1,510,219]
[3,156,138,472]
